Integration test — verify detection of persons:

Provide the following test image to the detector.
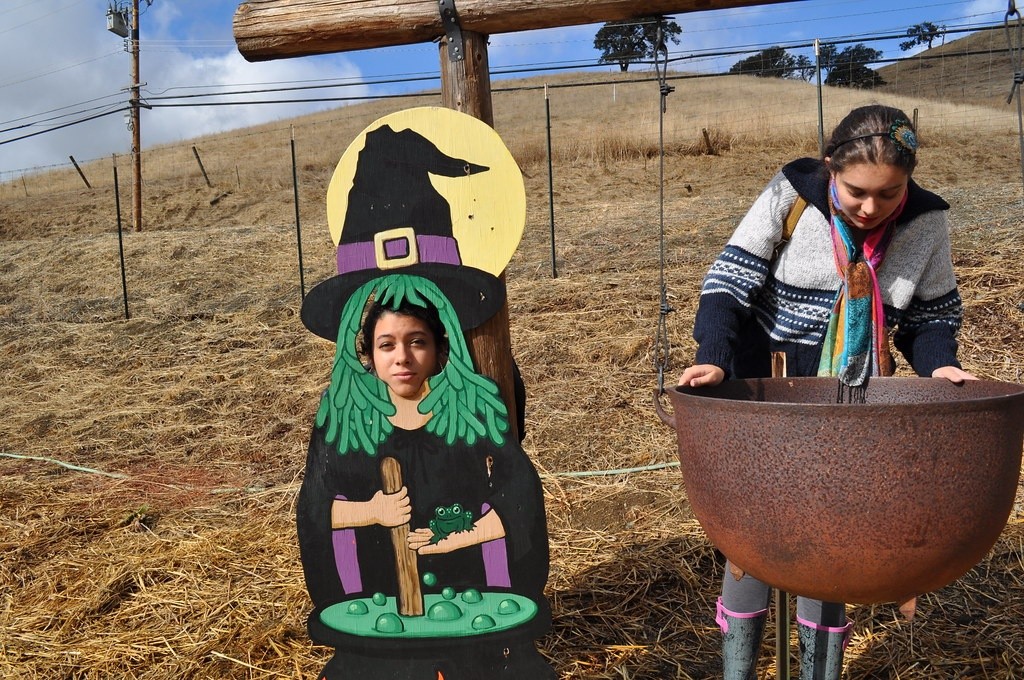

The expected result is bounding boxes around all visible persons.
[677,104,982,679]
[361,299,442,397]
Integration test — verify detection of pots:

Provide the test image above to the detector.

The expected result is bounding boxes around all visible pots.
[652,375,1023,603]
